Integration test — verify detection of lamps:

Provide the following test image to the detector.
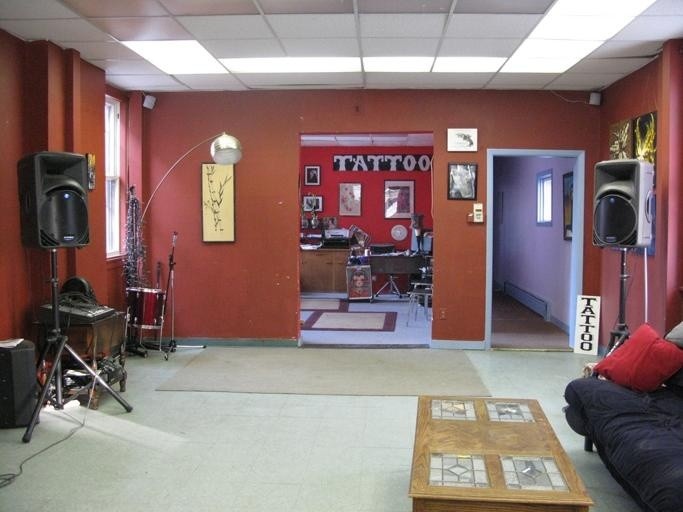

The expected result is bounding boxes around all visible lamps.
[141,131,243,225]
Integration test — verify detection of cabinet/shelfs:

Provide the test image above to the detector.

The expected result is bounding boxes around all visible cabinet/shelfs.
[299,249,350,292]
[31,312,129,410]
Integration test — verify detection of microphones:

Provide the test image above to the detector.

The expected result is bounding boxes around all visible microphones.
[172,232,178,248]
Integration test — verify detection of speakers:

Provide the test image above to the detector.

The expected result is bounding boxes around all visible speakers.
[0,339,40,429]
[589,91,602,105]
[17,152,90,249]
[142,96,158,110]
[592,158,654,248]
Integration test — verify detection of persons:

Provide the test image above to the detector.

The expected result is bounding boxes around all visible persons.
[348,270,371,298]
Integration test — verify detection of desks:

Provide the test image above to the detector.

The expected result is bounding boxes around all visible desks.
[408,396,594,512]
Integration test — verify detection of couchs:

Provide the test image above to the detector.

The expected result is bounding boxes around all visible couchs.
[563,331,682,511]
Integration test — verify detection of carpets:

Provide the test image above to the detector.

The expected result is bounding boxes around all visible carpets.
[154,348,492,398]
[301,298,349,312]
[301,311,398,331]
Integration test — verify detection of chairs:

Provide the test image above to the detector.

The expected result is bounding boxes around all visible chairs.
[406,283,432,326]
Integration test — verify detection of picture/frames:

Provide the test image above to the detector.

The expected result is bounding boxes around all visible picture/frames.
[202,164,235,243]
[303,195,323,212]
[304,165,321,186]
[384,180,415,220]
[563,172,573,240]
[448,163,477,200]
[339,183,362,216]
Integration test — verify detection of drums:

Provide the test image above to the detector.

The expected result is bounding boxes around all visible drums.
[125,285,164,331]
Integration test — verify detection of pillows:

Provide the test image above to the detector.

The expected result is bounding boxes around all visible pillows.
[594,323,683,393]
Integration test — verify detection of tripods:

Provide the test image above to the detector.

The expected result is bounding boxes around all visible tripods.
[23,253,132,443]
[593,252,630,378]
[160,254,206,360]
